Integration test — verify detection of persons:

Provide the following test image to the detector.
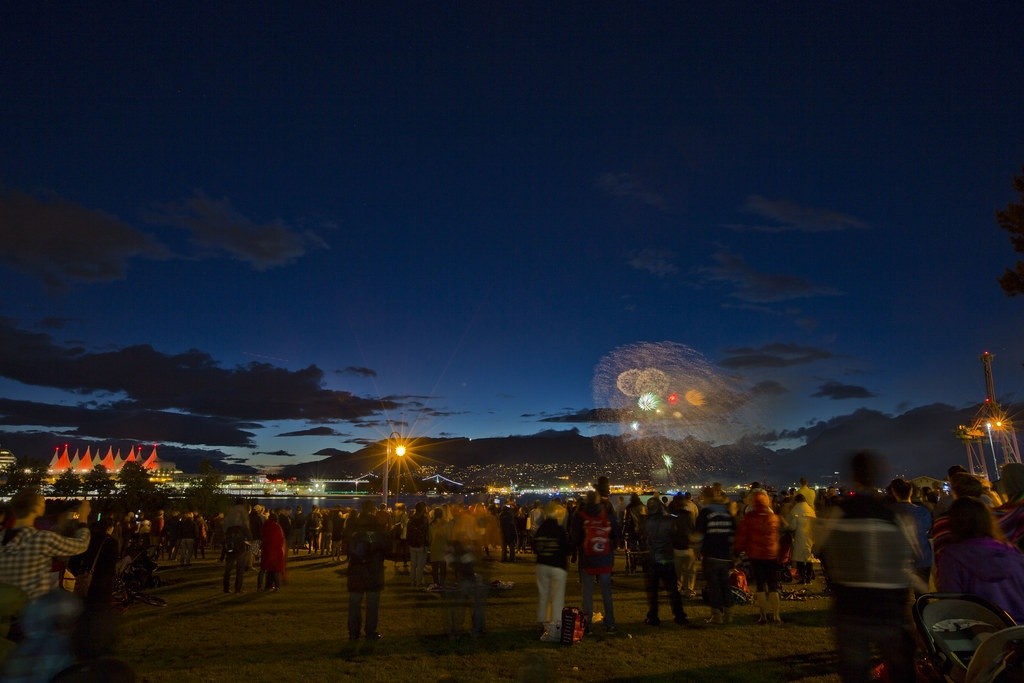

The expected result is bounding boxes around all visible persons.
[938,496,1024,627]
[891,478,933,660]
[68,517,118,603]
[532,503,573,640]
[43,505,75,591]
[116,508,226,566]
[696,484,738,624]
[0,486,91,642]
[734,494,788,621]
[570,489,617,636]
[253,464,1024,586]
[641,496,690,625]
[673,494,697,596]
[342,498,403,641]
[447,503,503,642]
[220,501,251,594]
[813,449,924,683]
[259,514,286,589]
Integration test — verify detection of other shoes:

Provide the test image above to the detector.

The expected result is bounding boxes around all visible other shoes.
[365,632,384,640]
[348,635,360,641]
[606,624,619,635]
[584,626,592,635]
[674,619,686,625]
[645,619,660,626]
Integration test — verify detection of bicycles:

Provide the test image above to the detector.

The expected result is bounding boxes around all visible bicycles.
[114,575,167,612]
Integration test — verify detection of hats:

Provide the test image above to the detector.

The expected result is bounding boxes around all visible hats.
[254,505,265,516]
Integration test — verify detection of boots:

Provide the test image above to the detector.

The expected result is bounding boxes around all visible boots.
[704,608,723,625]
[767,591,781,624]
[757,592,767,624]
[723,607,736,624]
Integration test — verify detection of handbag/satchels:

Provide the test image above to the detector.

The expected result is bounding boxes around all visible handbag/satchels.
[74,574,92,592]
[643,552,663,576]
[534,537,560,556]
[540,620,562,642]
[560,607,585,645]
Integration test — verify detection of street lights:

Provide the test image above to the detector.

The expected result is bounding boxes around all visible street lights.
[996,420,1018,465]
[986,423,1001,481]
[383,430,406,508]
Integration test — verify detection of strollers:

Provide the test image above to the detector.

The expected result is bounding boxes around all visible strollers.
[910,591,1024,683]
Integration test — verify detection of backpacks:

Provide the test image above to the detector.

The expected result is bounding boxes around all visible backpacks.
[577,508,612,557]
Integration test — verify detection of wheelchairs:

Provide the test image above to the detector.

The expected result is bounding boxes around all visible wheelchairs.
[626,535,651,576]
